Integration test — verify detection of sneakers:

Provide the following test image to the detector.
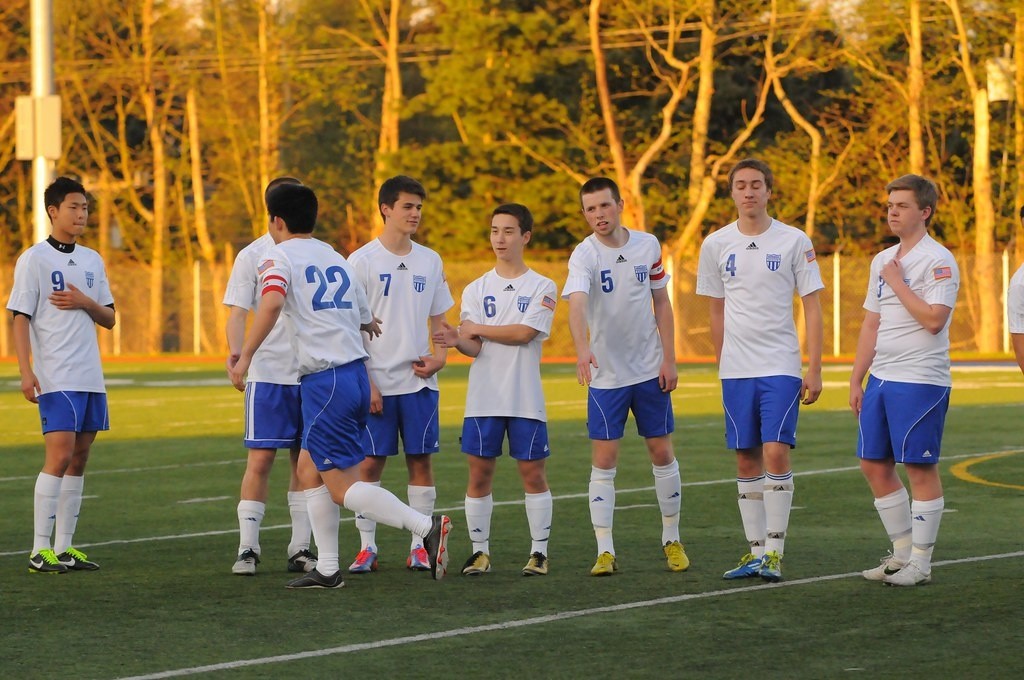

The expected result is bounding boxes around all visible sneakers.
[462,552,491,575]
[863,558,907,581]
[522,552,549,576]
[286,564,345,590]
[423,515,452,579]
[287,549,317,572]
[758,552,781,582]
[591,552,618,576]
[723,555,763,579]
[663,541,690,572]
[231,548,259,574]
[885,563,931,587]
[407,547,432,570]
[28,548,68,573]
[57,547,100,571]
[348,547,378,574]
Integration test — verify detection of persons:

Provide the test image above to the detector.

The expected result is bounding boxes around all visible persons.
[430,203,557,577]
[222,177,452,590]
[696,160,825,582]
[1008,206,1024,371]
[346,176,455,573]
[849,173,959,587]
[561,178,690,577]
[6,178,116,574]
